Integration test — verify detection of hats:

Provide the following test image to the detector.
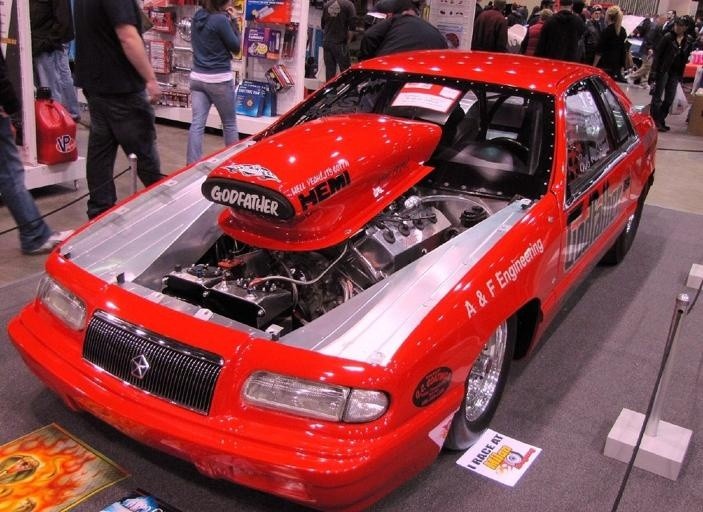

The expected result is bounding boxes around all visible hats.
[673,16,689,26]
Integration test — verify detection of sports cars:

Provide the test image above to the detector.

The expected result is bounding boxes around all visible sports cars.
[8,50,657,512]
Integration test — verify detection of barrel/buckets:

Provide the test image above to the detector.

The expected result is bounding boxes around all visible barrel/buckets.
[35,86,78,165]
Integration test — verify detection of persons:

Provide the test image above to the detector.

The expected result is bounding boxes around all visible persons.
[186,0,242,165]
[319,0,449,86]
[71,0,162,220]
[0,44,78,255]
[28,0,81,121]
[471,0,703,134]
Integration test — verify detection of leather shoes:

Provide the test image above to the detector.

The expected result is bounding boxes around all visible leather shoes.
[654,121,670,132]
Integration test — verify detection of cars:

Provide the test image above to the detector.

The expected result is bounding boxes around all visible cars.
[621,15,703,84]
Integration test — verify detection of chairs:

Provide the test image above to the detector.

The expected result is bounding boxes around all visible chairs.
[442,95,541,186]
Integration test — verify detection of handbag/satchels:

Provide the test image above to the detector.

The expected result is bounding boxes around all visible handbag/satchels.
[625,50,633,70]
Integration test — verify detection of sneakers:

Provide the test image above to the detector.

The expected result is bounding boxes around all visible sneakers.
[32,230,78,255]
